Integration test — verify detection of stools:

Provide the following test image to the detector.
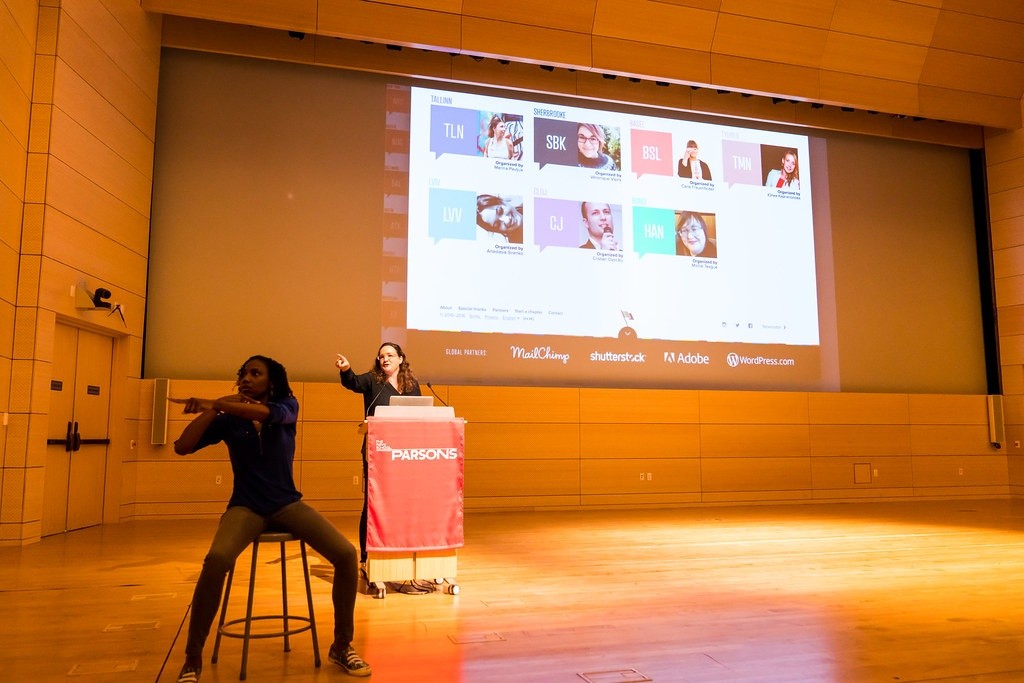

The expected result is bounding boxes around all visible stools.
[209,528,322,678]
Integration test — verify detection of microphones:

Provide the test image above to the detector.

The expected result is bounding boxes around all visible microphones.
[427,382,449,407]
[366,381,387,416]
[604,227,615,251]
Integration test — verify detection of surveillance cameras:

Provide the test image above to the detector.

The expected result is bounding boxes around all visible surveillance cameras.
[93,287,112,308]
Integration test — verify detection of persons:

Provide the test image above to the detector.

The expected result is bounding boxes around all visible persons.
[576,123,618,172]
[482,116,515,161]
[578,201,622,252]
[677,139,712,182]
[475,193,523,244]
[676,211,717,258]
[174,354,371,683]
[765,151,799,190]
[334,342,422,580]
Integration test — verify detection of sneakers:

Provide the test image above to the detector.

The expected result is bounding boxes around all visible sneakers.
[328,643,372,677]
[175,661,204,683]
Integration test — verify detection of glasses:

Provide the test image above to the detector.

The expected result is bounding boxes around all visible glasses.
[678,227,702,236]
[577,135,599,144]
[376,353,397,361]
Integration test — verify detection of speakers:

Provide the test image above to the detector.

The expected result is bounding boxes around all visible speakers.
[151,377,170,445]
[986,394,1006,444]
